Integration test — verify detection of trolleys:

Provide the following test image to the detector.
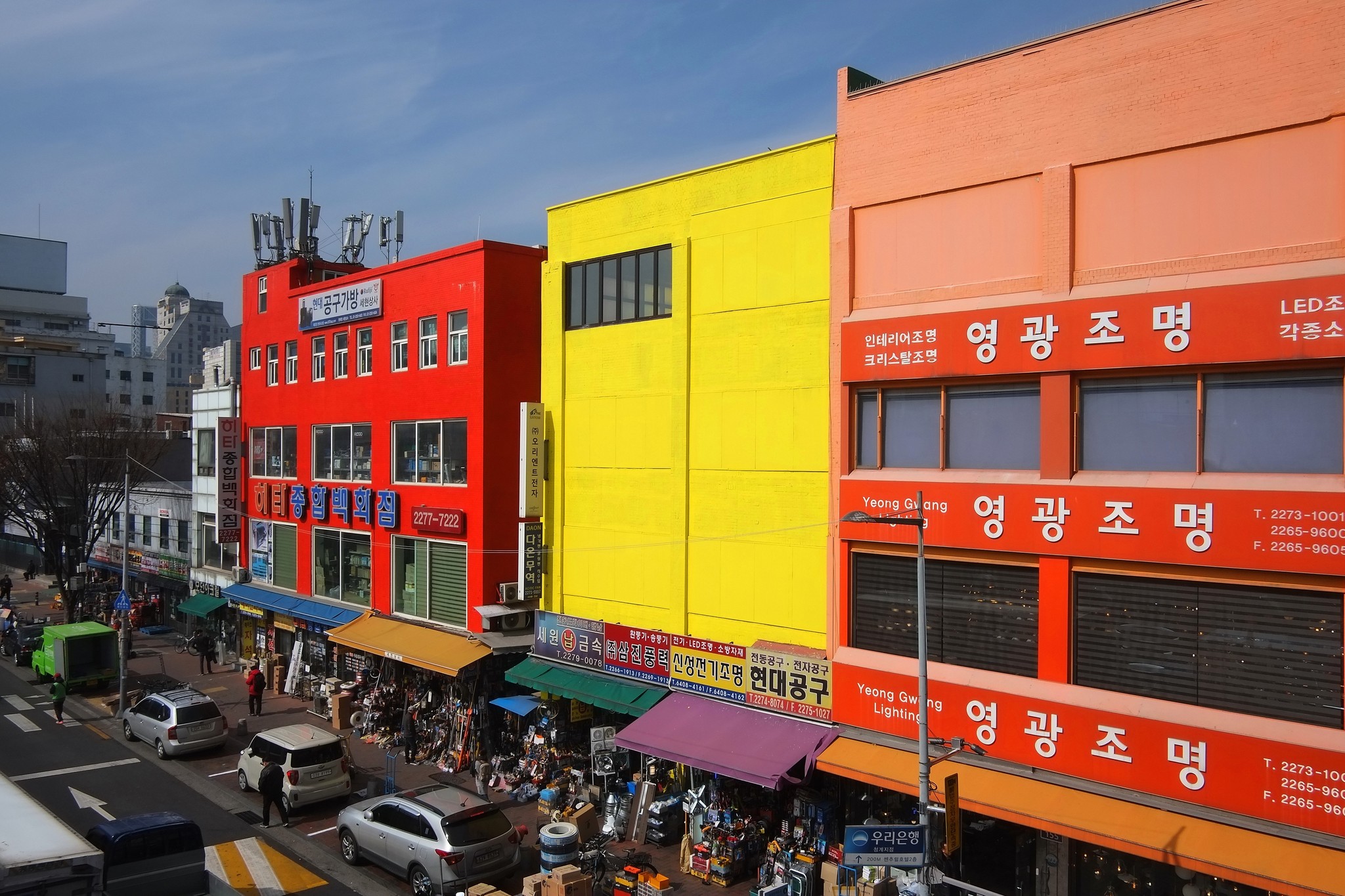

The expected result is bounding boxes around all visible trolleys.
[384,747,403,795]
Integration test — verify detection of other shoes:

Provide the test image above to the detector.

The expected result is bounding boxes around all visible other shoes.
[54,715,58,720]
[207,671,212,673]
[283,823,289,826]
[248,713,254,715]
[56,720,64,724]
[256,714,261,716]
[201,673,205,675]
[260,824,269,828]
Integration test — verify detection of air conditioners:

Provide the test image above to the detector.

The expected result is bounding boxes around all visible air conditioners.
[232,566,245,582]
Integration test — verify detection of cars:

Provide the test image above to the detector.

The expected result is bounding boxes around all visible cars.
[0,623,51,667]
[237,723,353,817]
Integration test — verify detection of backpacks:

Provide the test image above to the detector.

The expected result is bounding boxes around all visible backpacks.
[477,760,492,781]
[253,673,266,691]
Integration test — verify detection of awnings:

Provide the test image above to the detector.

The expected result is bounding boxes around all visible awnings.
[505,656,669,717]
[176,593,228,619]
[221,584,362,628]
[472,627,535,655]
[324,612,493,677]
[87,559,140,577]
[490,696,542,717]
[615,692,839,790]
[136,572,188,591]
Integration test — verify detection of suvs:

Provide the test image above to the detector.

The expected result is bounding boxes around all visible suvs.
[122,684,229,760]
[336,781,521,896]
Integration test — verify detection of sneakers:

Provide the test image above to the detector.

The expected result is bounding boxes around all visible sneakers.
[405,761,409,764]
[410,760,420,765]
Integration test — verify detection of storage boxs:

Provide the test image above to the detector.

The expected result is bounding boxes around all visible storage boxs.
[244,660,260,679]
[325,677,378,738]
[464,843,674,896]
[488,773,501,787]
[504,776,547,803]
[101,612,111,622]
[412,460,440,483]
[690,839,747,887]
[327,459,371,480]
[271,653,286,695]
[568,801,600,844]
[819,842,917,896]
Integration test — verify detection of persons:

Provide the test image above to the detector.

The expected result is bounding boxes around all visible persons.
[403,706,420,765]
[0,574,12,603]
[23,560,35,581]
[258,755,289,828]
[192,629,212,675]
[245,666,263,716]
[50,673,69,724]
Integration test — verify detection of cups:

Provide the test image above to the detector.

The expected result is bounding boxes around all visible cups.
[727,837,737,843]
[718,837,722,843]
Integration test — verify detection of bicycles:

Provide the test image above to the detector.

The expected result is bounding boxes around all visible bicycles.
[175,632,200,657]
[583,841,658,883]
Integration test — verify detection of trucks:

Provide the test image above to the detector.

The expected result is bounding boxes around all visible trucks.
[30,620,121,694]
[0,768,106,896]
[84,811,248,896]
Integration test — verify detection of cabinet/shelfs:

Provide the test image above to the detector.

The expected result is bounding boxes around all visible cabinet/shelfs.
[397,456,452,483]
[320,456,371,481]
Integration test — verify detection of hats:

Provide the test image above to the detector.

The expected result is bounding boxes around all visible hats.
[52,673,61,678]
[408,706,419,711]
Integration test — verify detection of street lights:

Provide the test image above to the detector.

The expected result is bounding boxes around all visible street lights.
[842,490,938,895]
[63,447,133,718]
